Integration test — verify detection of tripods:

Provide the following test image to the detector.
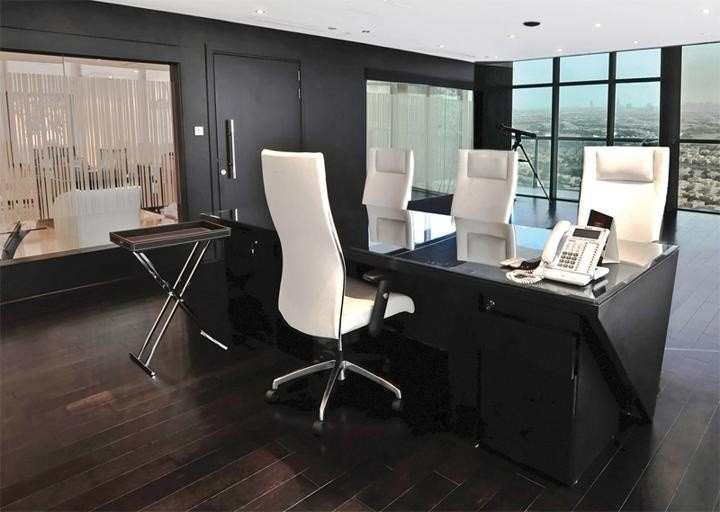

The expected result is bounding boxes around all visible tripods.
[504,137,553,205]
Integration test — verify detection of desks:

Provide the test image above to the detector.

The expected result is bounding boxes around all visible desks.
[199,184,680,491]
[108,217,234,379]
[0,219,48,257]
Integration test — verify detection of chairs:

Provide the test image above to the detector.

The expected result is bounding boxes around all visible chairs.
[358,146,417,210]
[449,147,521,224]
[256,147,421,443]
[575,146,672,241]
[453,216,519,265]
[52,184,142,252]
[364,204,417,256]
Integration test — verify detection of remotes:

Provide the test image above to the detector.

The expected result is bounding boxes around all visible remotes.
[499,258,541,269]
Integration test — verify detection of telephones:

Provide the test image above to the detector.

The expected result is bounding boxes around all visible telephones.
[533,219,610,287]
